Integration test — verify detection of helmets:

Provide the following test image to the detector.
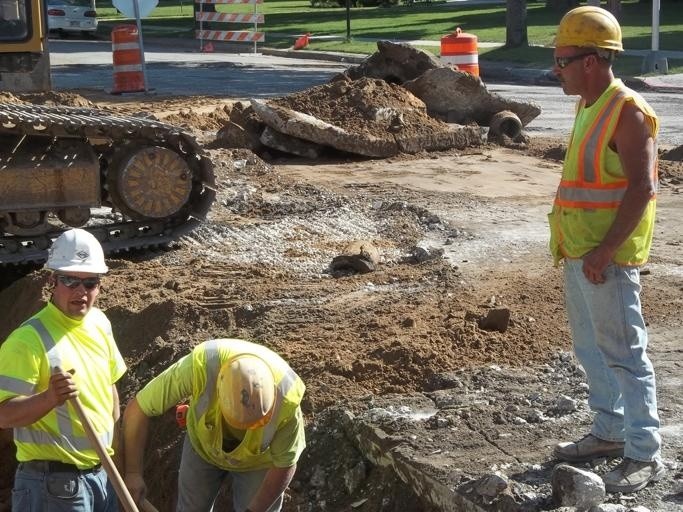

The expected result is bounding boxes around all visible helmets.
[555,6,624,51]
[44,228,108,275]
[216,354,277,430]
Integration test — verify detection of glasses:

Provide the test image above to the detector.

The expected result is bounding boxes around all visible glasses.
[54,275,100,289]
[551,53,598,69]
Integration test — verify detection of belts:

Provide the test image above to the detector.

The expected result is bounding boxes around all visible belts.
[27,460,94,473]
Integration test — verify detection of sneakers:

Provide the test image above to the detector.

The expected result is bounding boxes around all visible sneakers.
[554,434,625,463]
[603,457,666,493]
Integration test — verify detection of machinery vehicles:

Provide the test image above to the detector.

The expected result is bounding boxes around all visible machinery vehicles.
[0,0,218,270]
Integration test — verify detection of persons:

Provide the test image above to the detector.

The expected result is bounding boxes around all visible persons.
[122,339,305,511]
[549,6,662,493]
[1,228,125,511]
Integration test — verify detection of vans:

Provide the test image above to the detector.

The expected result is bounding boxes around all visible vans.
[45,0,99,42]
